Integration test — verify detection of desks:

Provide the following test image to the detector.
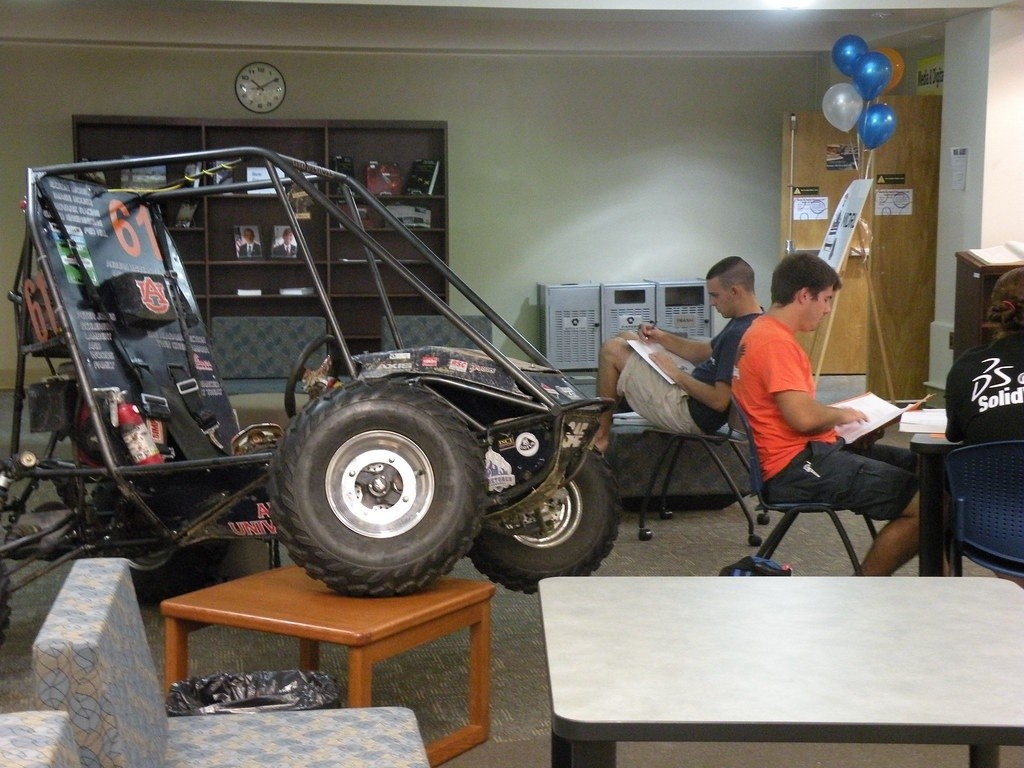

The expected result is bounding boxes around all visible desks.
[159,561,494,768]
[909,432,963,577]
[538,568,1024,768]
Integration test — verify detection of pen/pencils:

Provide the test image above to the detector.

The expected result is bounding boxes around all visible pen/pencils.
[646,319,658,342]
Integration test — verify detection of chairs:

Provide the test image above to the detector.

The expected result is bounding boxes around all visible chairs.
[945,438,1024,577]
[0,557,434,768]
[206,316,328,438]
[637,388,877,576]
[382,314,494,356]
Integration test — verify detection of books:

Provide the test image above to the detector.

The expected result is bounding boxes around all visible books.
[627,340,696,384]
[968,240,1024,266]
[827,391,937,445]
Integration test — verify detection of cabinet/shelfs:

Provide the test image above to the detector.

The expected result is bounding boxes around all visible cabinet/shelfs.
[72,113,451,375]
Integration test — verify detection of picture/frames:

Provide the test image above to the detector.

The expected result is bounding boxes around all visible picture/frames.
[231,223,267,261]
[268,223,304,260]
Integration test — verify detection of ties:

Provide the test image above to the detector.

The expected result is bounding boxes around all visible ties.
[286,246,289,255]
[248,245,252,255]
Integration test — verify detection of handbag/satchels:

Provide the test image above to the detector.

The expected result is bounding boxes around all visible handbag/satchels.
[719,556,792,576]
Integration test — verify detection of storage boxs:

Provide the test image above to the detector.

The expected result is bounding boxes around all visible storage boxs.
[338,201,432,229]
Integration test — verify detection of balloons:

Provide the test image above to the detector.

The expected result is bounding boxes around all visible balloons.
[858,105,897,149]
[876,48,905,93]
[822,82,863,133]
[853,50,892,102]
[831,34,869,77]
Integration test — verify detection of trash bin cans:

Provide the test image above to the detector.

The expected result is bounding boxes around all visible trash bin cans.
[538,279,711,370]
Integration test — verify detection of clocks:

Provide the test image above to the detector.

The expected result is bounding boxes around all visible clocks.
[235,61,287,114]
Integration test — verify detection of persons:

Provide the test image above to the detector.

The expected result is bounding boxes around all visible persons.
[731,252,920,577]
[273,228,296,256]
[239,228,260,257]
[592,256,767,456]
[943,267,1024,587]
